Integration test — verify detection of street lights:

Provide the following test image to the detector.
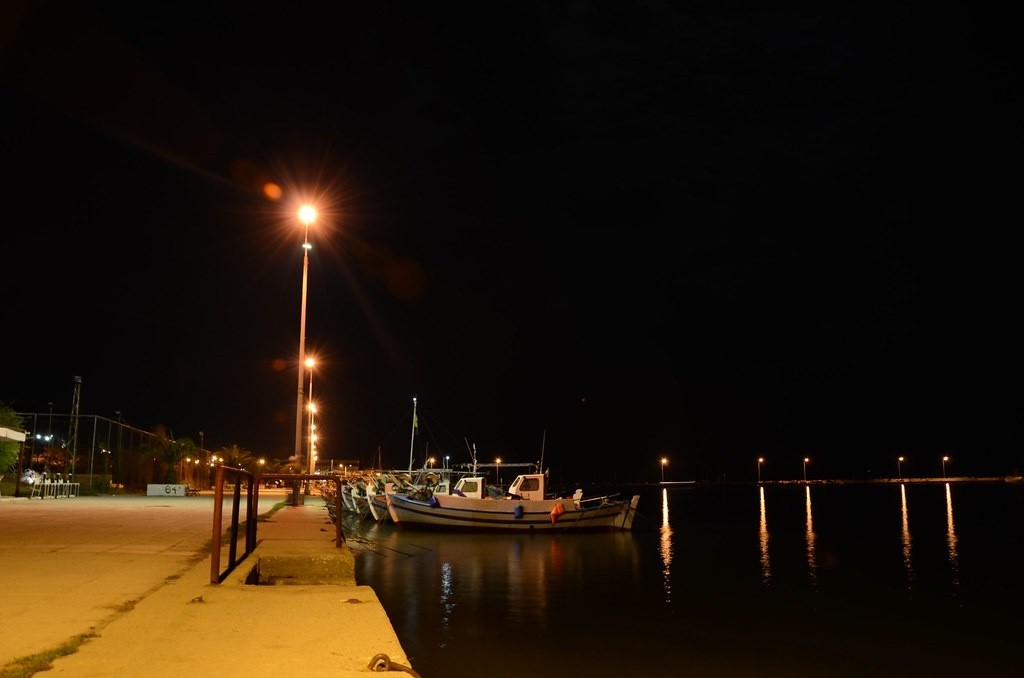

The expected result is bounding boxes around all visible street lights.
[662,459,666,482]
[309,403,317,475]
[116,410,120,451]
[292,198,320,507]
[758,458,764,480]
[303,355,316,495]
[898,456,904,478]
[942,457,949,478]
[199,430,204,450]
[48,402,54,437]
[804,457,809,480]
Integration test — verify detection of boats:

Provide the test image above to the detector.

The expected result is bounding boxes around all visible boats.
[341,397,640,535]
